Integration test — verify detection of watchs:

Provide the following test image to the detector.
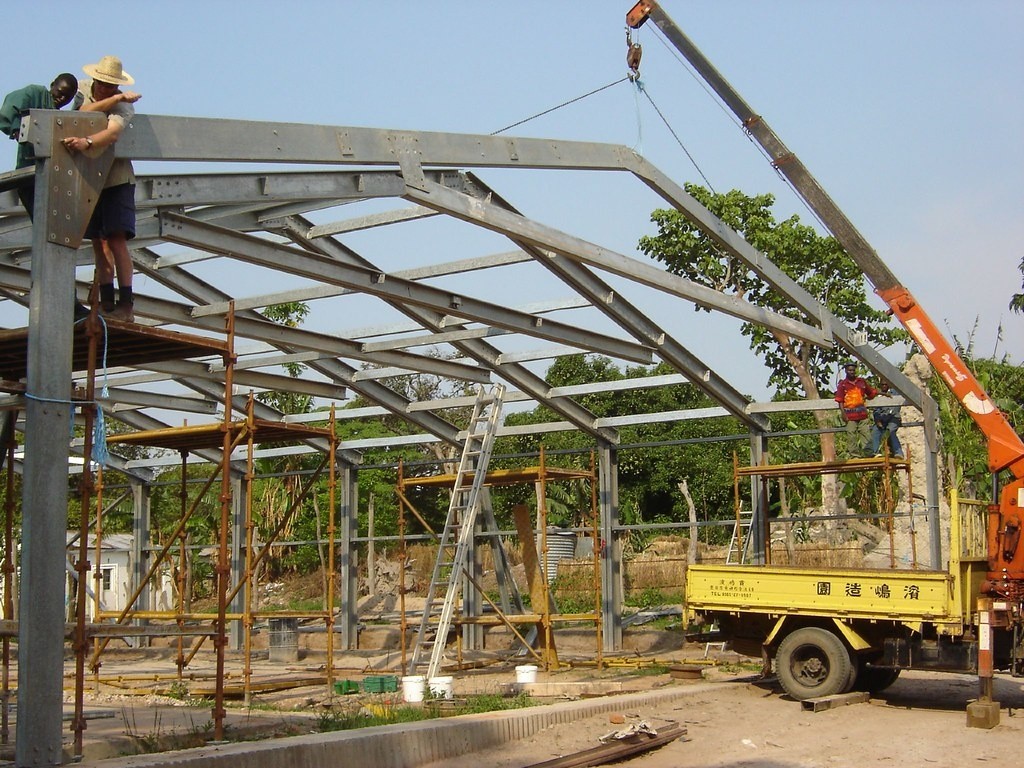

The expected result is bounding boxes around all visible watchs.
[85,136,92,149]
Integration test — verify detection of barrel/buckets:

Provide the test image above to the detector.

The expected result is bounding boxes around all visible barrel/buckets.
[515,666,538,682]
[402,676,425,702]
[429,676,453,699]
[534,535,574,584]
[268,618,298,662]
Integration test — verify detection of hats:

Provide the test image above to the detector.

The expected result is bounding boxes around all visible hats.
[845,362,857,367]
[83,56,135,85]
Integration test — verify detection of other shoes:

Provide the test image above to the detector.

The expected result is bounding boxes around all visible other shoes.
[894,454,904,460]
[102,300,135,323]
[98,299,114,316]
[871,451,883,458]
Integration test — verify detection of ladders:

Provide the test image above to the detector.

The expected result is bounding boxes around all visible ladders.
[704,499,753,656]
[408,382,508,681]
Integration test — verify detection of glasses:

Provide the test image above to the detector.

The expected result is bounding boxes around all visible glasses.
[95,79,118,89]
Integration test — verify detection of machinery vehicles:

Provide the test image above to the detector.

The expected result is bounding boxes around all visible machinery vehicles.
[625,0,1024,702]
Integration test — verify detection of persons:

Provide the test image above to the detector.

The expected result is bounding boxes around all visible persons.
[0,73,92,320]
[59,56,142,324]
[835,362,892,460]
[869,377,905,460]
